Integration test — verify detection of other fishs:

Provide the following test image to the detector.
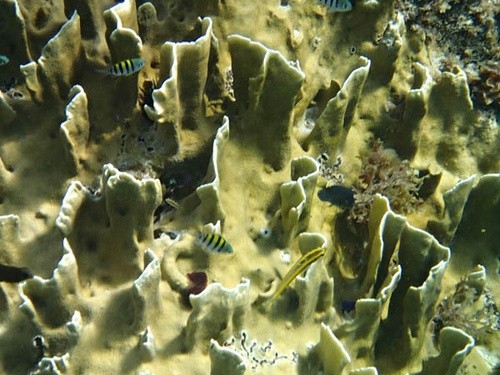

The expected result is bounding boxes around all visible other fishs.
[95,57,146,77]
[0,55,10,66]
[317,0,352,12]
[271,247,326,300]
[185,227,234,254]
[318,186,358,210]
